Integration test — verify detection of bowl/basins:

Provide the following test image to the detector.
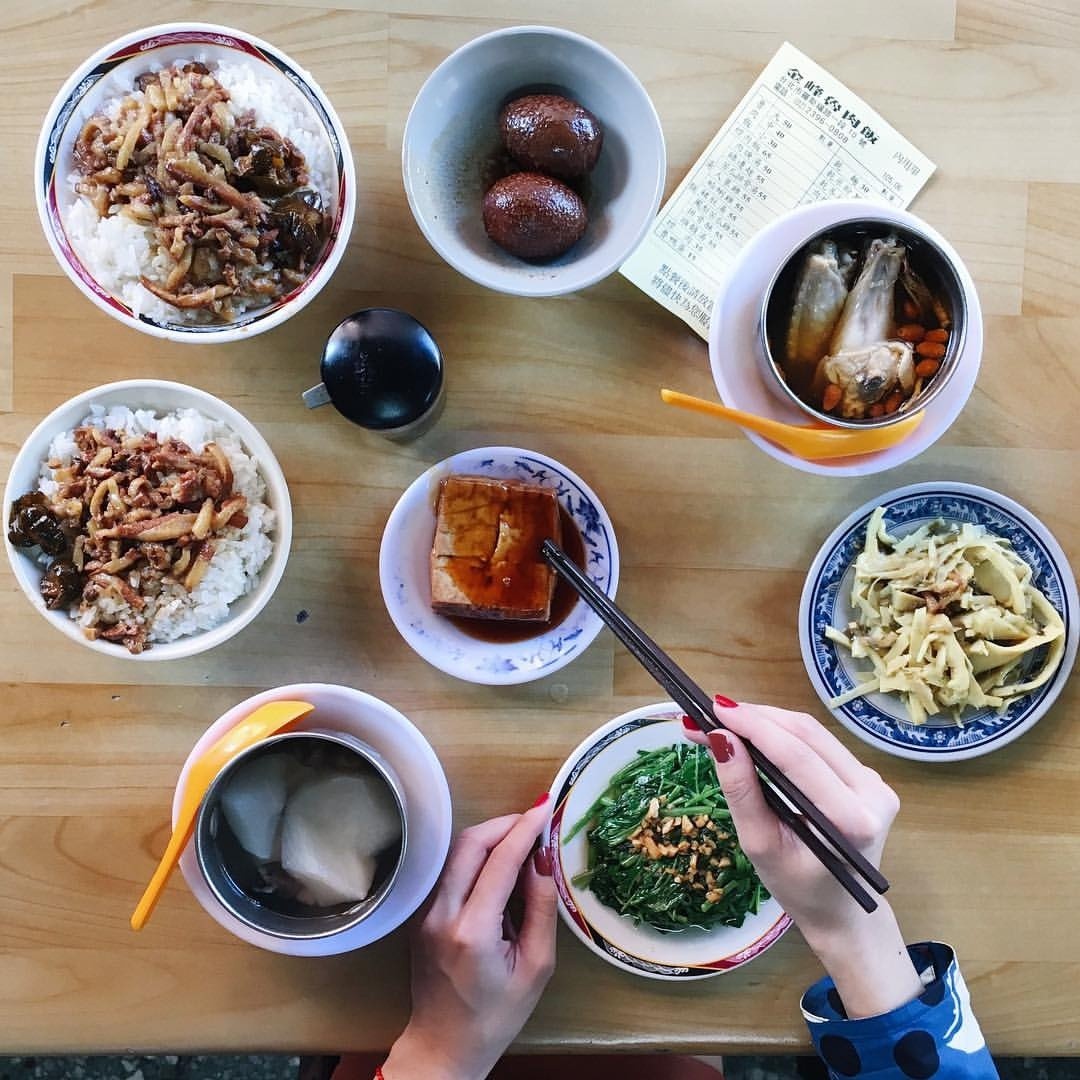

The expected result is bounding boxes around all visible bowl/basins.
[170,684,454,959]
[33,23,358,345]
[403,25,668,301]
[753,216,970,429]
[1,378,291,662]
[197,730,413,940]
[377,444,621,686]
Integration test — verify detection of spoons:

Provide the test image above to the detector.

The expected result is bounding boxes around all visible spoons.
[126,699,316,930]
[658,388,927,460]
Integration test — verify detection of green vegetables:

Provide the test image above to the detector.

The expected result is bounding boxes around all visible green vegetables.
[561,741,772,934]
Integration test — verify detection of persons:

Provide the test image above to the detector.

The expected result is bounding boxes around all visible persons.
[331,694,1007,1080]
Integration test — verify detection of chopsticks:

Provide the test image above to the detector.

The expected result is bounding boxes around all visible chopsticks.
[541,533,889,914]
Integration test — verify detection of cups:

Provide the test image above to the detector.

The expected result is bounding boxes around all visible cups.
[302,306,444,437]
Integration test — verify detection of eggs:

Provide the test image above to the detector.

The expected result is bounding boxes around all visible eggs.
[483,172,587,261]
[499,94,603,174]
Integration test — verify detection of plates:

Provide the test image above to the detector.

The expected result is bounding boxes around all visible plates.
[542,703,802,980]
[798,480,1079,762]
[710,196,984,480]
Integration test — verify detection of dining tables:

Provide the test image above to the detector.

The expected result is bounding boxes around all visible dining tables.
[0,3,1080,1067]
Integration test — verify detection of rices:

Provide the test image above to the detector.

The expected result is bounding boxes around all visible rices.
[36,403,275,645]
[66,53,331,328]
